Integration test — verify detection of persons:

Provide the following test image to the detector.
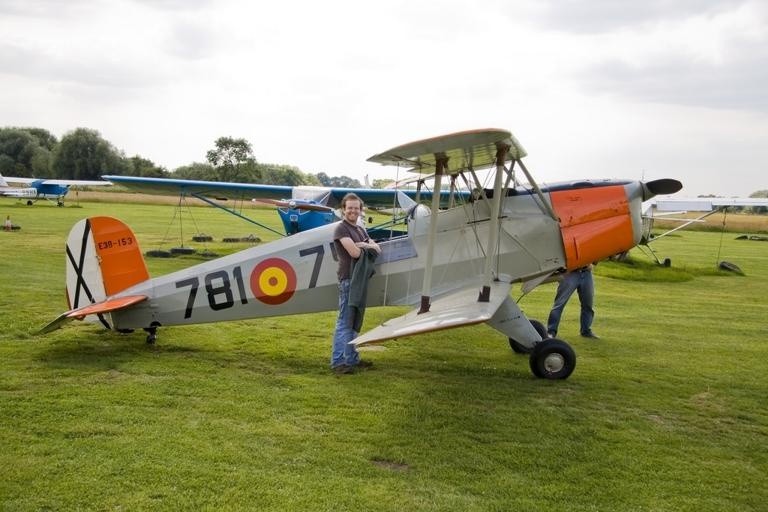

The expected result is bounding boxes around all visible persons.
[330,193,382,375]
[547,263,600,339]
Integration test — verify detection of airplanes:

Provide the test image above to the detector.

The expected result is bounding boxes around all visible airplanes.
[31,128,684,382]
[593,197,768,268]
[100,176,471,238]
[0,173,115,207]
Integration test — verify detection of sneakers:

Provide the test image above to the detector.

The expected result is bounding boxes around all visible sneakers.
[353,361,373,367]
[581,332,600,339]
[329,364,353,374]
[547,332,554,339]
